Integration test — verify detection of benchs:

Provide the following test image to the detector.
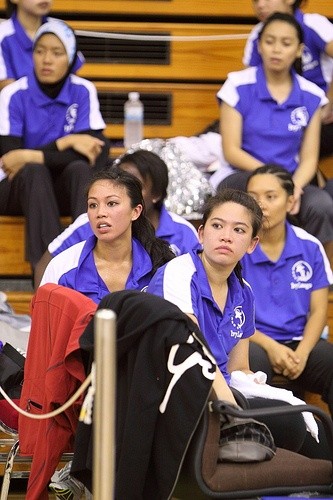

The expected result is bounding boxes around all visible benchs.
[1,0,333,276]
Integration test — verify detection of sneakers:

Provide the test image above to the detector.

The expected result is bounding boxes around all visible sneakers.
[48,476,84,500]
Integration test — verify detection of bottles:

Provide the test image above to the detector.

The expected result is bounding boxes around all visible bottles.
[122,91,144,150]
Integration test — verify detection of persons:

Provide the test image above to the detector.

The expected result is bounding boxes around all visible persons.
[240,163,333,421]
[38,168,175,306]
[217,0,333,245]
[35,149,200,287]
[0,0,107,272]
[147,188,262,405]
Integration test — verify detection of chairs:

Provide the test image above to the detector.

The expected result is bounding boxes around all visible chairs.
[0,398,78,499]
[18,284,98,500]
[96,289,333,500]
